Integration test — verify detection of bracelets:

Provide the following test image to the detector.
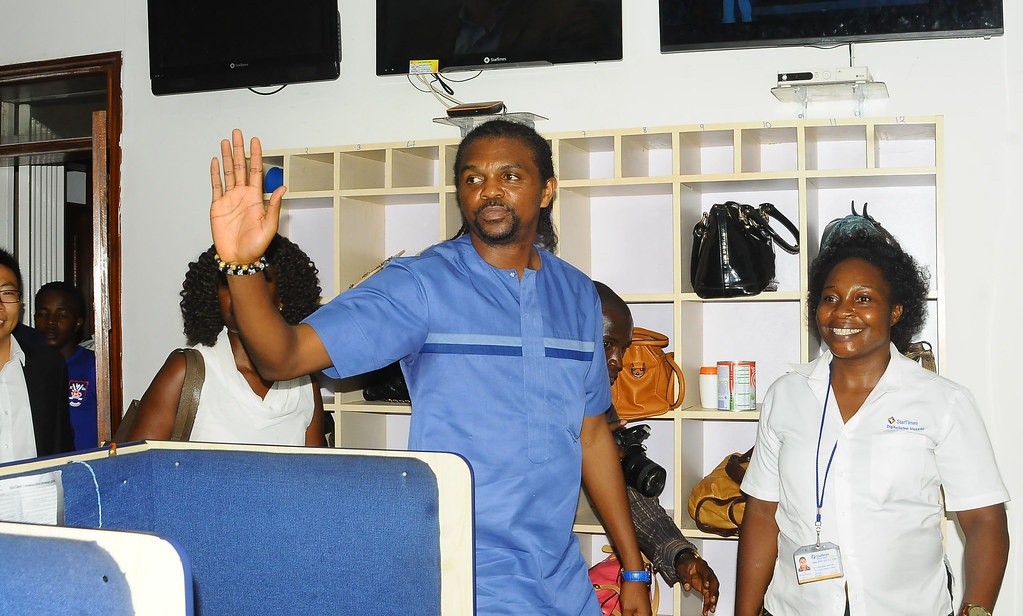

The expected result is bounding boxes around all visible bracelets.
[214,252,269,276]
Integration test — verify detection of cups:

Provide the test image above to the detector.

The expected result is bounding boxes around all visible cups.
[699,367,718,408]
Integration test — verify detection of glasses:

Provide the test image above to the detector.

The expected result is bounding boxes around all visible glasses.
[0,290,22,303]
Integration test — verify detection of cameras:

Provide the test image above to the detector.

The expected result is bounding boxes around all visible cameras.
[612,423,667,498]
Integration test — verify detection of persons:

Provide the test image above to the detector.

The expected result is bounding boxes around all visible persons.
[0,247,76,466]
[122,233,325,448]
[798,557,810,572]
[595,283,720,616]
[209,118,652,616]
[734,217,1009,616]
[34,281,99,452]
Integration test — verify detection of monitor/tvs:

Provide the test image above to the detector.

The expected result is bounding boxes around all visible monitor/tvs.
[148,0,340,97]
[377,0,623,75]
[658,0,1005,55]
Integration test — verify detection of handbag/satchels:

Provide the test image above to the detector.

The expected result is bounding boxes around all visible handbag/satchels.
[110,348,205,444]
[687,446,755,536]
[610,327,685,421]
[588,544,659,616]
[691,201,800,298]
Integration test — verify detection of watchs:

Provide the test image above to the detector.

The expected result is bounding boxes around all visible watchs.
[959,602,991,616]
[622,563,652,590]
[674,548,701,570]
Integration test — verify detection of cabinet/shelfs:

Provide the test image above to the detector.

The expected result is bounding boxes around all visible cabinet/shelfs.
[245,114,945,616]
